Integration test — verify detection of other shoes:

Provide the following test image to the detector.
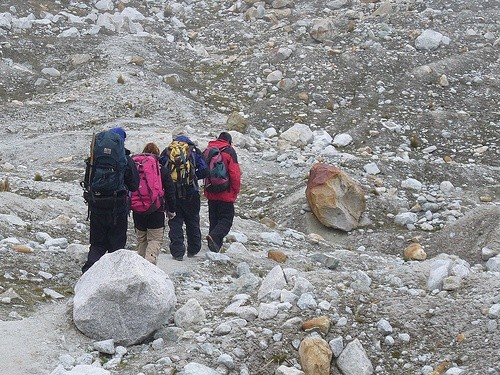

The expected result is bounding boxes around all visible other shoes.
[187,252,193,258]
[206,235,219,252]
[173,256,183,261]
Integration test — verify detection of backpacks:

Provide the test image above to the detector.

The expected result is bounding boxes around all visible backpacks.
[166,140,198,186]
[127,152,165,213]
[203,145,230,193]
[82,129,132,215]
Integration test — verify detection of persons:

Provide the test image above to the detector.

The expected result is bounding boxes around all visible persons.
[132,142,177,266]
[202,131,241,253]
[159,127,207,260]
[81,127,140,273]
